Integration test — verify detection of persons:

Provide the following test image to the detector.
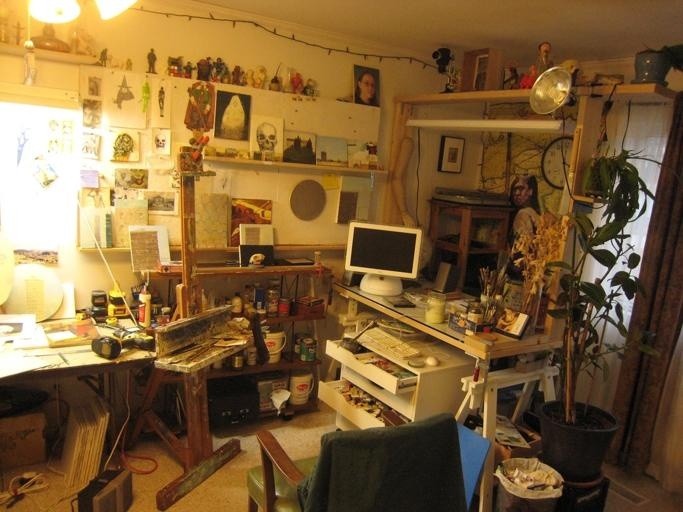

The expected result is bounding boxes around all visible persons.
[536,41,553,76]
[506,172,541,280]
[495,313,517,329]
[147,48,157,73]
[355,70,378,106]
[518,65,539,90]
[157,85,165,119]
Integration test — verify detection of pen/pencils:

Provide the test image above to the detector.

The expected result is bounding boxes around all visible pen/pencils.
[484,301,497,322]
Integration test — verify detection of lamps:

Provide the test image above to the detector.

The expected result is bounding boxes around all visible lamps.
[529,65,577,116]
[27,0,139,51]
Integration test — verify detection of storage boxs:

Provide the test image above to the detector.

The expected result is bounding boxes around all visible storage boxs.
[473,423,543,496]
[0,406,47,470]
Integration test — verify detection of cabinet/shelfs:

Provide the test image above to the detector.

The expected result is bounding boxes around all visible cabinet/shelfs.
[427,189,514,293]
[317,271,562,434]
[194,155,389,252]
[154,260,330,436]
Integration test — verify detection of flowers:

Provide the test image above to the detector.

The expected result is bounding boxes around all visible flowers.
[513,216,575,278]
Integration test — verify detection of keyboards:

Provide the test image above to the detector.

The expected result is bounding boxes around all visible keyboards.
[362,327,419,360]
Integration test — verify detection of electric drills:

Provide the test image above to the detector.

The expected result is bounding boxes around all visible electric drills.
[91,329,155,360]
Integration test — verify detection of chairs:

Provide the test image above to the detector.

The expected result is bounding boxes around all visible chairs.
[246,411,468,511]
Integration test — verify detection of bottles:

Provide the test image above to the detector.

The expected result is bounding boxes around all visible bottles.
[230,291,242,313]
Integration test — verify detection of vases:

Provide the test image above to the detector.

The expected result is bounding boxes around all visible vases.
[517,276,543,336]
[634,49,673,82]
[540,211,660,484]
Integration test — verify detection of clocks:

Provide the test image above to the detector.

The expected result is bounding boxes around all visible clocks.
[541,137,574,189]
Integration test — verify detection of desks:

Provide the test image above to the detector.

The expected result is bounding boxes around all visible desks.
[0,315,155,469]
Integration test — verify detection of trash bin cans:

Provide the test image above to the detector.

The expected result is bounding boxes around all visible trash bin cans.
[495,457,566,512]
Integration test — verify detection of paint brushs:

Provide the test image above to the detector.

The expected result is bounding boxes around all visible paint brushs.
[161,340,218,364]
[480,264,508,298]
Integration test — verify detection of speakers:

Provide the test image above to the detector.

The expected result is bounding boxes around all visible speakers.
[434,262,459,293]
[339,267,358,288]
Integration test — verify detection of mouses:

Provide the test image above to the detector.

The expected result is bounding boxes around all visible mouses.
[427,356,439,367]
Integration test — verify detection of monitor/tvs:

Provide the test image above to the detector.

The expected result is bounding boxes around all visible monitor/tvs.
[345,222,422,294]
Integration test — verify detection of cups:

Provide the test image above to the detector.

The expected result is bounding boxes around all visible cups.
[423,292,447,325]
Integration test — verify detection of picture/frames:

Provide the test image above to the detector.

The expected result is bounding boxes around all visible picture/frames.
[474,53,489,90]
[438,136,465,174]
[353,64,381,106]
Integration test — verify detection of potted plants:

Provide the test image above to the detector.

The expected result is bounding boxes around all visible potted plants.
[580,145,683,223]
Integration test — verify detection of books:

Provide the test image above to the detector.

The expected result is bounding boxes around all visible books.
[238,244,274,266]
[239,223,274,245]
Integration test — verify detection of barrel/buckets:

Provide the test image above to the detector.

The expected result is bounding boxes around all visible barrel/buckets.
[289,371,314,405]
[264,331,286,357]
